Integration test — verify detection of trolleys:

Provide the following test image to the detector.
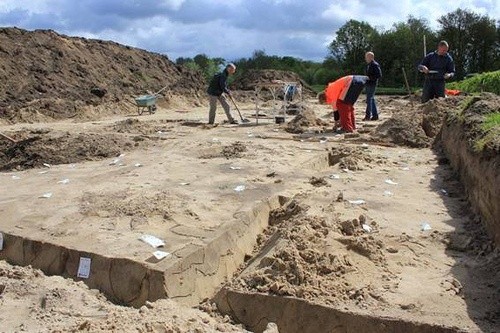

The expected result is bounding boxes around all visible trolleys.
[134,93,164,115]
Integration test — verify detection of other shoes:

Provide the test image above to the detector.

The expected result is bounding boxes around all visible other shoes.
[337,127,348,133]
[362,116,371,121]
[371,116,378,120]
[231,121,238,124]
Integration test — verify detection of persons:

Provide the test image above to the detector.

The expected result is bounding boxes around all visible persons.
[417,40,456,104]
[361,52,382,121]
[317,75,366,132]
[206,63,238,124]
